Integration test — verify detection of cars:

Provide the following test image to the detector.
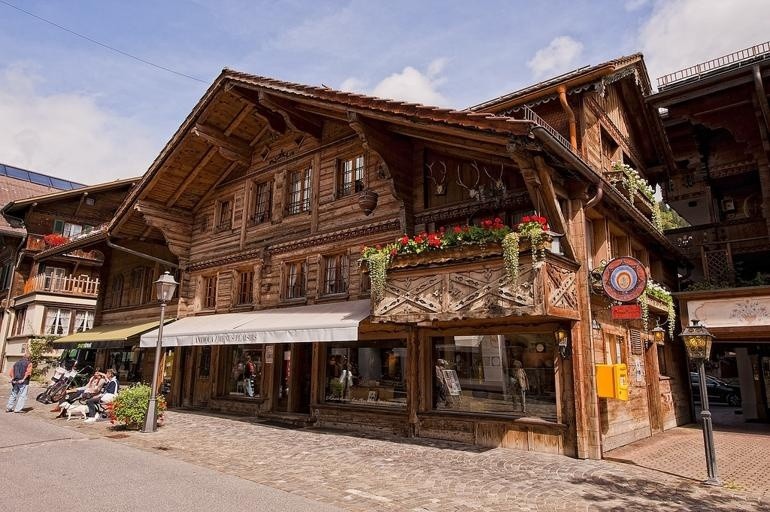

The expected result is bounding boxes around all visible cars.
[689,370,743,406]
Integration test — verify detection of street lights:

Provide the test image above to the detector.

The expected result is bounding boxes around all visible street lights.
[140,270,179,433]
[680,316,723,488]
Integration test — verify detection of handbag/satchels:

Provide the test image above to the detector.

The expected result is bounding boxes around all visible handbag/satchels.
[101,393,115,402]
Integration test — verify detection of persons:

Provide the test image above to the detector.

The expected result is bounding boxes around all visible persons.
[339,361,359,399]
[86,369,120,423]
[510,359,530,414]
[49,367,108,418]
[436,356,452,409]
[6,351,33,414]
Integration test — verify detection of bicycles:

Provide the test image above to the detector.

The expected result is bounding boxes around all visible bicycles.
[36,365,93,404]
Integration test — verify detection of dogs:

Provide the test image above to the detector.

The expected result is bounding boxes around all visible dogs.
[59,401,90,422]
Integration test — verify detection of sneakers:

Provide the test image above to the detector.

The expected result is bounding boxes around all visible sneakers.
[50,407,60,412]
[5,409,26,413]
[85,412,99,423]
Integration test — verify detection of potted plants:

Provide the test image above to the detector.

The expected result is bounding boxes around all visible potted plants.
[106,380,167,429]
